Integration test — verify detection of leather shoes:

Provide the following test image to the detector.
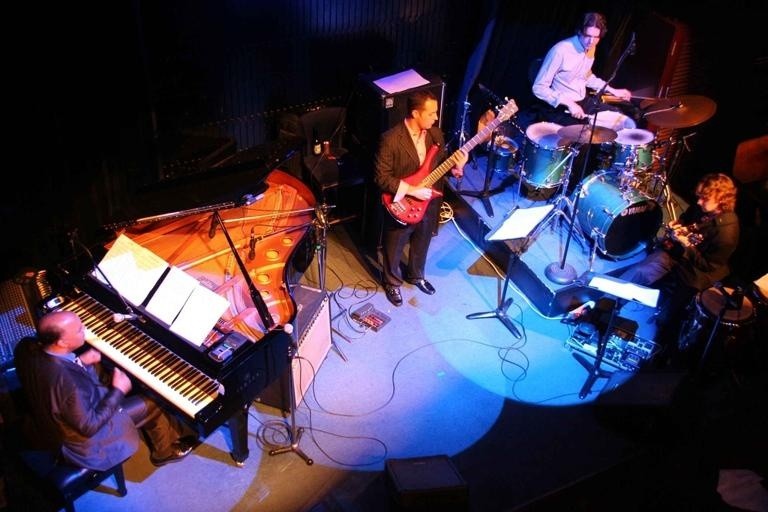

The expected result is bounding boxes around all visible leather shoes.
[417,278,436,295]
[384,286,403,306]
[149,442,192,466]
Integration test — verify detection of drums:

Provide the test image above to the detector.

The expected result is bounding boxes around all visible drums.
[518,121,567,189]
[678,286,755,350]
[612,129,653,169]
[589,110,636,152]
[570,170,662,260]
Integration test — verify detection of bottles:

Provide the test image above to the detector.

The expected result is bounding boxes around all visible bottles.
[312,133,329,156]
[283,325,296,360]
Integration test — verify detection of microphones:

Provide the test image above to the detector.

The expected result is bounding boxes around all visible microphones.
[113,312,136,324]
[284,323,299,350]
[713,282,738,307]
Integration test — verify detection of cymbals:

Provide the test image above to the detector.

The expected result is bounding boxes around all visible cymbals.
[644,94,716,128]
[557,124,617,144]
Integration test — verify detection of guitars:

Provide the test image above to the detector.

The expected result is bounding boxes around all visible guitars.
[380,97,518,225]
[660,229,702,249]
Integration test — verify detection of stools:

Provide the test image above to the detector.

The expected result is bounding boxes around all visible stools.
[675,284,755,360]
[49,455,131,512]
[747,273,768,311]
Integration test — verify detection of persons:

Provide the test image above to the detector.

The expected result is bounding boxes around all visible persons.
[608,171,740,309]
[27,310,193,470]
[533,12,633,125]
[375,91,469,307]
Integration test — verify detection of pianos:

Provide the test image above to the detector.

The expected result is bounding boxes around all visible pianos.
[39,170,318,463]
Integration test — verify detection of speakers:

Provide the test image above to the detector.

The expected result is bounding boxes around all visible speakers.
[595,371,675,418]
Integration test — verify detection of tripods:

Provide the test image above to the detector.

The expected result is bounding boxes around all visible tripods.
[461,154,505,217]
[466,256,523,340]
[572,318,611,396]
[269,354,313,465]
